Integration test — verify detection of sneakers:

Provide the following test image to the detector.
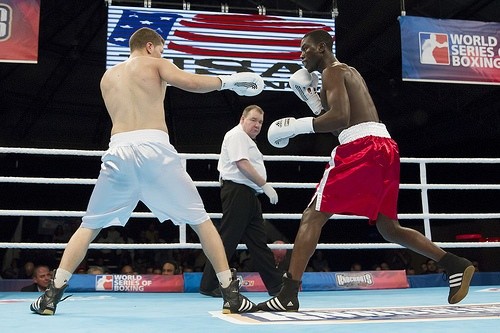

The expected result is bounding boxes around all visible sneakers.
[218,268,258,313]
[437,251,475,304]
[30,269,73,315]
[258,272,302,312]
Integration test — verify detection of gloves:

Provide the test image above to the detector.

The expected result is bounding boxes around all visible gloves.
[289,68,325,116]
[217,71,265,96]
[260,183,278,204]
[267,116,315,148]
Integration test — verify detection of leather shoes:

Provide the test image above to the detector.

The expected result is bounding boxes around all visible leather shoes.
[268,280,284,296]
[201,284,223,297]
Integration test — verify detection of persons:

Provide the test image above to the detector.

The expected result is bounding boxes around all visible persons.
[200,105,284,297]
[0,217,480,293]
[257,29,475,312]
[30,27,264,315]
